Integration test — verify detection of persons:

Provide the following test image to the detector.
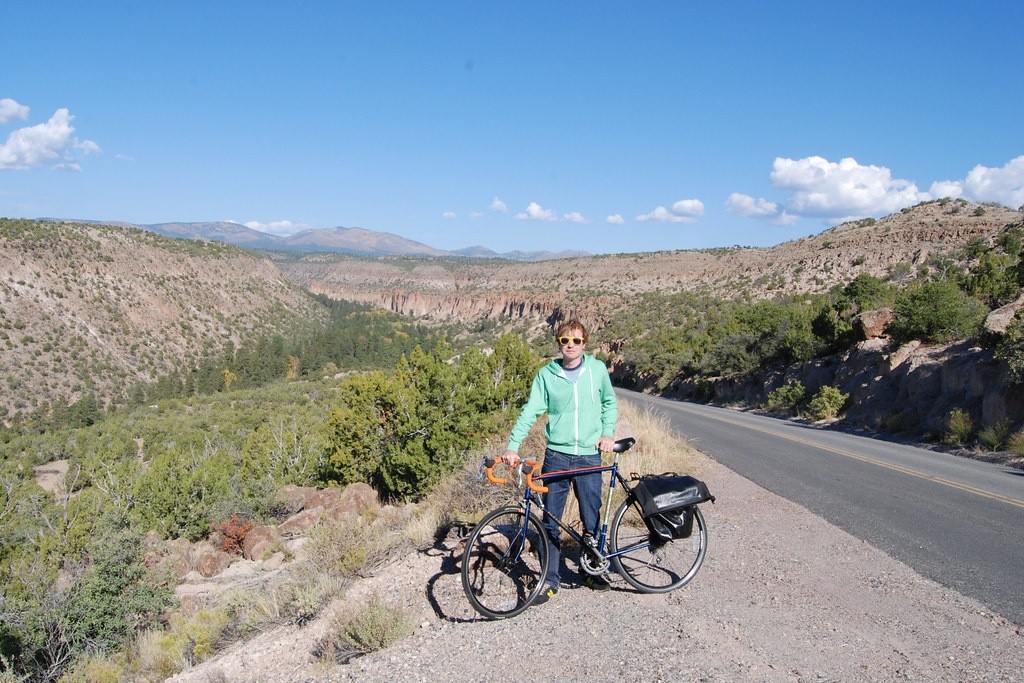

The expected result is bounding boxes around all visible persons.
[500,319,619,606]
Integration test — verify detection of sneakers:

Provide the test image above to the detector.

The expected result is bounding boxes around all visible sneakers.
[578,566,611,592]
[529,583,558,608]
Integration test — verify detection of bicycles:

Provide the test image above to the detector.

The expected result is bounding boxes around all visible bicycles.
[460,437,708,621]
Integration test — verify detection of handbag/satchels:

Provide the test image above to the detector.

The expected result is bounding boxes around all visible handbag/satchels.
[631,472,716,549]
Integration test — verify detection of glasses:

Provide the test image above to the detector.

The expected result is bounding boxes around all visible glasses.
[559,336,585,345]
